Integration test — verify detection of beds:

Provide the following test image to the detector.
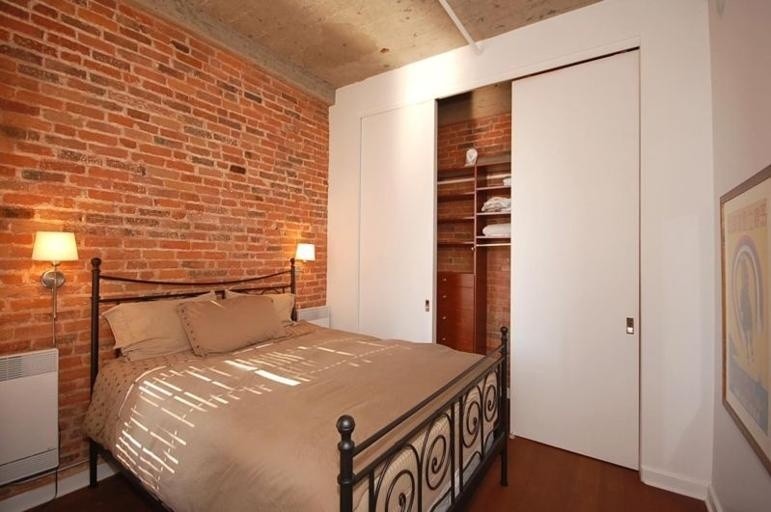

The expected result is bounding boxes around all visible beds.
[90,258,507,512]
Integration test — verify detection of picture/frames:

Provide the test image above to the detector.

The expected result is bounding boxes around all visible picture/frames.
[720,164,771,477]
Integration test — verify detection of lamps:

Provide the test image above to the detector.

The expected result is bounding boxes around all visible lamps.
[31,231,79,346]
[295,243,316,263]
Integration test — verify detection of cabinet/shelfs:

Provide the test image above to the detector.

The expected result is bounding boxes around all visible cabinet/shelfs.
[438,161,511,356]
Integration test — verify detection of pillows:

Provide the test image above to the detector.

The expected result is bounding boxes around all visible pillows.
[100,289,298,363]
